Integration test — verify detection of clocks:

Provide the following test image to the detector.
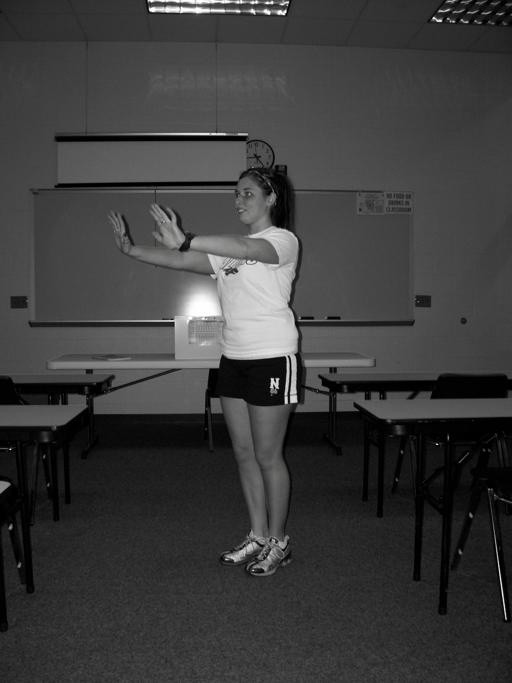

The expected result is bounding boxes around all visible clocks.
[244,137,275,171]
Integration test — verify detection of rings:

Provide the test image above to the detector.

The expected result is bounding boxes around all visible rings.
[161,218,166,225]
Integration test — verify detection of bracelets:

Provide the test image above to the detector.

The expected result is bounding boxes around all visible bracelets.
[178,229,196,252]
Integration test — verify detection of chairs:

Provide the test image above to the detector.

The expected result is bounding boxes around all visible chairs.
[452,468,510,623]
[391,371,507,495]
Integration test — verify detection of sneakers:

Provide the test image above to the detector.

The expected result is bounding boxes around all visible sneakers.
[245,536,295,576]
[218,531,268,566]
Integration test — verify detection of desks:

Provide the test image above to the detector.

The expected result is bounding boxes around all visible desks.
[47,348,376,458]
[318,368,512,518]
[0,372,115,633]
[352,397,511,618]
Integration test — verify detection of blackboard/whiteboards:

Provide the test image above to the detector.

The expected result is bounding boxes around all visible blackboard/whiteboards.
[28,187,415,326]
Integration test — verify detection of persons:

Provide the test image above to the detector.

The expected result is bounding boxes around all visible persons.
[108,166,306,579]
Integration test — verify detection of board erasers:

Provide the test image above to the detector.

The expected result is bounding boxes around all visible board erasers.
[325,316,341,320]
[299,316,314,320]
